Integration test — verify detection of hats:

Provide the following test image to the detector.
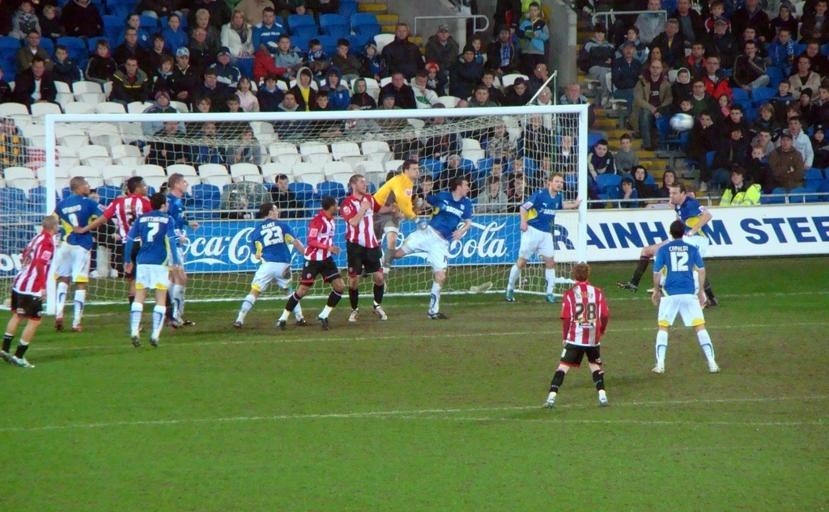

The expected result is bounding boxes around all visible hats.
[216,46,231,58]
[175,46,191,58]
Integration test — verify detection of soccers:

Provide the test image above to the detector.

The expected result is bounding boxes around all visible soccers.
[669,112,693,132]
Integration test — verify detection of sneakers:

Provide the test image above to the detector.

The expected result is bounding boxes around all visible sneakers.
[596,391,609,407]
[704,287,718,306]
[0,348,35,369]
[505,293,516,303]
[165,314,185,329]
[318,313,329,331]
[232,311,248,330]
[426,310,447,321]
[348,307,360,322]
[150,332,160,347]
[55,315,64,332]
[276,319,288,330]
[130,329,142,348]
[71,318,83,332]
[382,265,391,275]
[294,314,308,326]
[614,280,638,294]
[371,304,388,321]
[651,365,665,374]
[540,397,556,408]
[709,364,720,373]
[545,293,556,304]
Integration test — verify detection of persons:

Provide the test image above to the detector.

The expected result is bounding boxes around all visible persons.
[382,174,476,322]
[614,181,719,313]
[504,173,584,307]
[339,173,401,326]
[649,218,722,378]
[231,201,311,327]
[274,195,348,330]
[540,262,612,409]
[369,158,429,294]
[48,175,109,333]
[1,1,828,220]
[72,176,172,333]
[122,192,188,350]
[161,170,200,332]
[46,187,110,288]
[0,213,62,371]
